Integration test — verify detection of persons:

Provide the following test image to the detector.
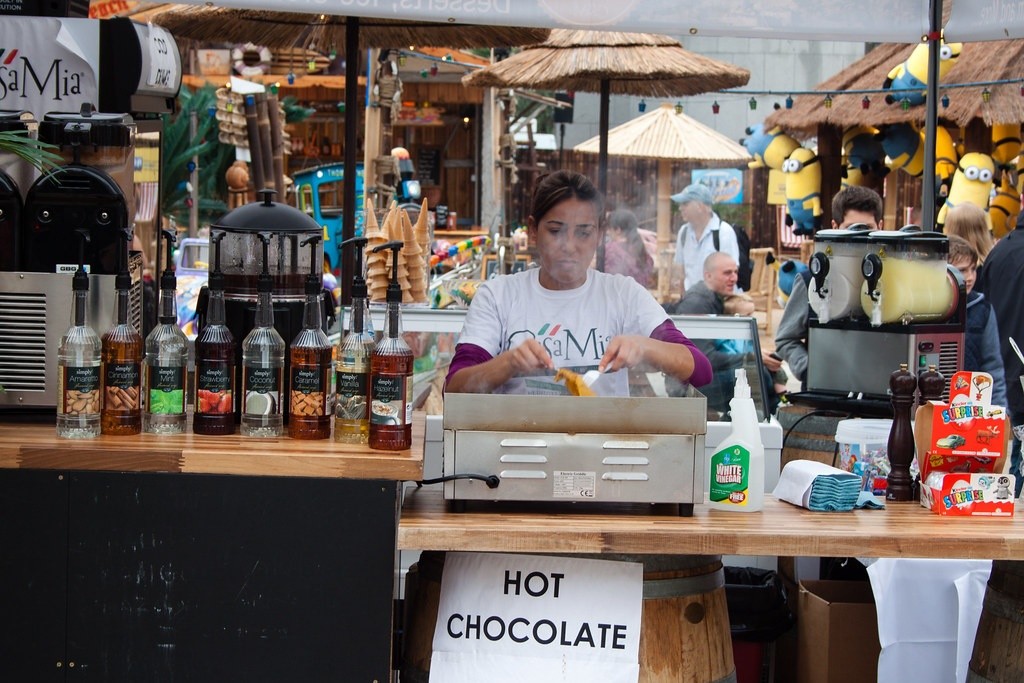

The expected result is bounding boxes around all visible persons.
[441,169,714,424]
[594,183,793,425]
[773,185,884,394]
[931,201,1024,500]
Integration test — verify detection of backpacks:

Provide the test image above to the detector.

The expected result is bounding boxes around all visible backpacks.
[680,222,751,292]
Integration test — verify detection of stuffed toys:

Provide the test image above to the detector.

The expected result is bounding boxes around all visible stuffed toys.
[881,27,963,107]
[765,251,811,310]
[840,114,1024,242]
[781,147,824,236]
[736,123,802,174]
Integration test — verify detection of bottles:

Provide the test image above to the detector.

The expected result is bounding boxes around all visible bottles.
[334,276,377,444]
[288,273,334,440]
[709,368,765,513]
[142,269,189,434]
[240,274,285,438]
[368,281,414,450]
[436,204,457,230]
[55,270,102,440]
[101,271,142,435]
[512,225,529,253]
[193,270,238,435]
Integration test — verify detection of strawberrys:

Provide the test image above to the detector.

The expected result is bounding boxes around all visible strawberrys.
[198,389,232,414]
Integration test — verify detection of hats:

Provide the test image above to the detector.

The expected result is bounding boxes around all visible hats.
[670,183,714,205]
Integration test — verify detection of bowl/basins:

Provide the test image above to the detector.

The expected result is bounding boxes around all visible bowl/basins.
[834,418,919,504]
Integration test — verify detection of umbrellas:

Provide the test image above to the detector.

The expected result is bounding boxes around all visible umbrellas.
[460,28,751,271]
[150,0,550,312]
[571,101,756,298]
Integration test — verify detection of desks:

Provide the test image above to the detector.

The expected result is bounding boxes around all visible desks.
[434,229,488,245]
[397,485,1024,560]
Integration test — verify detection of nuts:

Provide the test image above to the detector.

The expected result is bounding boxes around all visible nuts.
[66,389,101,415]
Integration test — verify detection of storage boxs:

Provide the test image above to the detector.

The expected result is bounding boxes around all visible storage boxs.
[914,370,1016,518]
[796,579,881,683]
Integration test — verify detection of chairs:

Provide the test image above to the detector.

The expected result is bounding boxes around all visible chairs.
[747,248,778,336]
